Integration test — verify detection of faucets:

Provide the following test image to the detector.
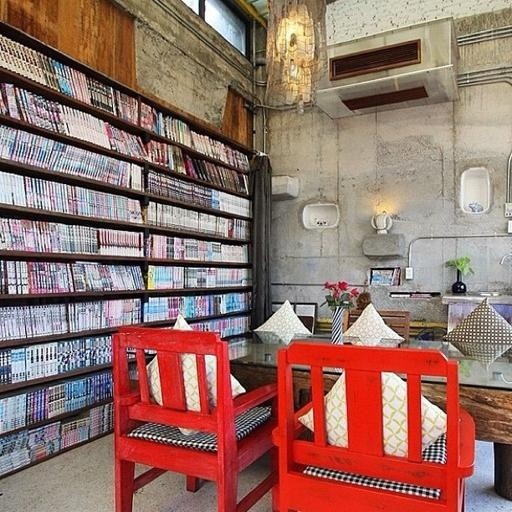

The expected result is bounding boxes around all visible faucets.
[499,253,512,265]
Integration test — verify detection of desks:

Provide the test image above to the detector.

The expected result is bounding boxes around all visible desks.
[220,331,512,501]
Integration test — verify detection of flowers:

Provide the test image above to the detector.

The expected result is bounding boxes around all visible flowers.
[322,281,360,308]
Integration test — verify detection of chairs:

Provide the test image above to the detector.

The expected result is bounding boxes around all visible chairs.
[268,302,318,335]
[111,326,299,512]
[343,309,410,340]
[271,341,475,512]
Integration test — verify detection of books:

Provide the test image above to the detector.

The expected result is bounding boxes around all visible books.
[0,35,257,484]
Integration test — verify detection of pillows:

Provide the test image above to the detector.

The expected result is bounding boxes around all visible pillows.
[342,303,406,347]
[298,368,447,458]
[443,297,512,369]
[137,315,246,436]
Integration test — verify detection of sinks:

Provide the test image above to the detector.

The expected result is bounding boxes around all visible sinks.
[477,292,512,297]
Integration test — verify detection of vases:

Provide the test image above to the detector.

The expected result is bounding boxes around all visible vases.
[330,305,350,345]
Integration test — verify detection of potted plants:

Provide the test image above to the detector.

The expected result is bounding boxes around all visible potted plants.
[445,257,475,294]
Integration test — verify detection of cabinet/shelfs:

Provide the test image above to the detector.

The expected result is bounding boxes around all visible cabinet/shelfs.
[0,21,261,480]
